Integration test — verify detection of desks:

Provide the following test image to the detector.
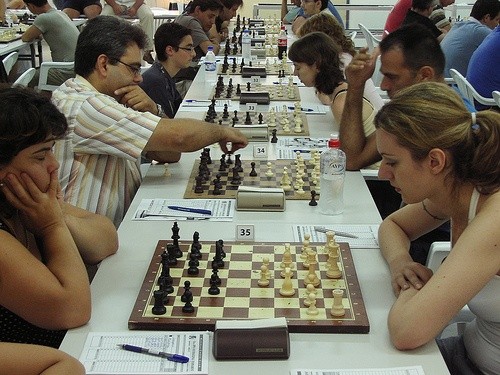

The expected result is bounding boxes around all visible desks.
[70,19,86,29]
[0,28,43,75]
[57,32,451,375]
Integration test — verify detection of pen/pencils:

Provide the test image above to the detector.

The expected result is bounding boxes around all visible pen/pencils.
[288,107,313,111]
[186,100,211,102]
[314,226,358,238]
[294,150,322,153]
[273,82,297,85]
[116,343,189,363]
[168,206,211,215]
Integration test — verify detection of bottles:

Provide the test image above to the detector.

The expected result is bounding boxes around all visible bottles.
[277,26,287,60]
[205,46,217,83]
[316,139,346,215]
[242,29,251,60]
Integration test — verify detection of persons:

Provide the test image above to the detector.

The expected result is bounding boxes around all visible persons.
[100,0,155,65]
[284,0,345,35]
[298,12,385,112]
[341,26,451,264]
[288,32,386,170]
[374,83,500,375]
[21,0,80,87]
[50,15,248,230]
[139,23,196,119]
[175,0,243,81]
[0,87,119,375]
[381,0,455,41]
[441,0,500,111]
[63,0,102,19]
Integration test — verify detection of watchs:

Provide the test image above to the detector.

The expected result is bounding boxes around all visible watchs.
[157,104,165,118]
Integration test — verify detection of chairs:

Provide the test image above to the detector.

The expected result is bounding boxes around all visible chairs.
[348,23,500,270]
[2,14,175,93]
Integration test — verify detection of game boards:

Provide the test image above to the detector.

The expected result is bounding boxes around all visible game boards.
[233,22,281,27]
[217,63,294,76]
[7,24,33,33]
[127,241,372,334]
[202,111,310,136]
[0,35,23,44]
[183,160,322,199]
[208,85,300,101]
[229,36,278,45]
[233,28,281,34]
[215,46,279,57]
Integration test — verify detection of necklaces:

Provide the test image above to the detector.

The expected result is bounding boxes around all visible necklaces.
[4,217,30,249]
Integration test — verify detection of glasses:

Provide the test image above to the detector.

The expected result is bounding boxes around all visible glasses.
[108,55,141,74]
[179,47,194,53]
